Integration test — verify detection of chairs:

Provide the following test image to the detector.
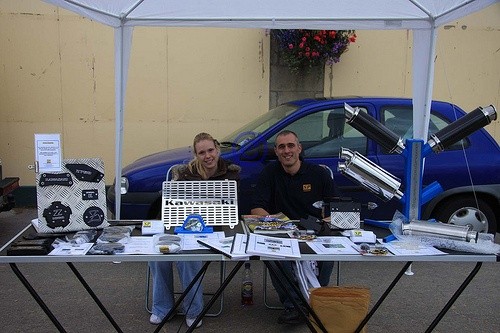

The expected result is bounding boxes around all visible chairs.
[319,111,345,143]
[263,165,341,310]
[146,164,225,317]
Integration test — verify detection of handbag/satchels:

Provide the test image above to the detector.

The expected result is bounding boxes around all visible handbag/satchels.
[307,285,371,333]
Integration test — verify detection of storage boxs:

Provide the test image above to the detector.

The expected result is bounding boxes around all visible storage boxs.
[309,286,371,333]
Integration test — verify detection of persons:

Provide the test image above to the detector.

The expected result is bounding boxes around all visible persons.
[149,132,241,328]
[250,130,334,324]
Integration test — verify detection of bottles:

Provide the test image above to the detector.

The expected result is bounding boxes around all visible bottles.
[241,262,254,306]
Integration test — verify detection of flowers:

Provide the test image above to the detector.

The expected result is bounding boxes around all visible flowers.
[271,29,356,78]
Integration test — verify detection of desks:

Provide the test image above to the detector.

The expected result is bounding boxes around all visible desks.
[244,221,500,333]
[0,219,250,333]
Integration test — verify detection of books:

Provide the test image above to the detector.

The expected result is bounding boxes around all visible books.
[197,231,302,260]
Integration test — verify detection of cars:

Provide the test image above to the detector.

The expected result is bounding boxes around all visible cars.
[107,95,500,255]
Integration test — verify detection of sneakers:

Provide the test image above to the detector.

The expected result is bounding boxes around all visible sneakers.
[186,316,202,328]
[278,304,305,325]
[150,311,176,324]
